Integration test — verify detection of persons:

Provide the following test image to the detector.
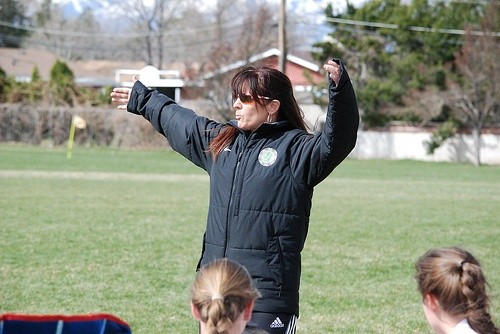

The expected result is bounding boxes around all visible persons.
[190,258,259,334]
[413,246,500,334]
[110,59,359,334]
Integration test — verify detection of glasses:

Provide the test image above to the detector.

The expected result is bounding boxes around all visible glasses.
[232,92,275,105]
